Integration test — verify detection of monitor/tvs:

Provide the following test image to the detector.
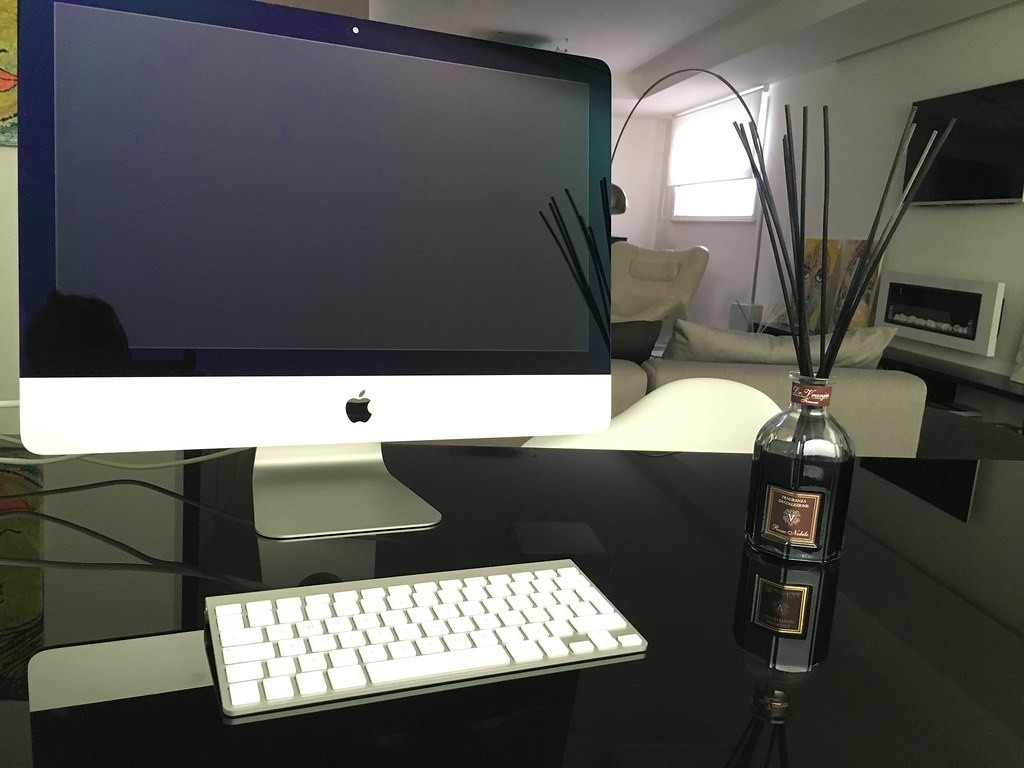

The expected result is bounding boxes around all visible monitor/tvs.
[28,533,580,768]
[859,457,982,525]
[16,0,613,538]
[902,79,1024,207]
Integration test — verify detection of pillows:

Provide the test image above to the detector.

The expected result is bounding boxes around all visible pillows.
[661,320,897,370]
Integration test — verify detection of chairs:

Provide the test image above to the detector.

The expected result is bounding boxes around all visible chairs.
[610,240,709,321]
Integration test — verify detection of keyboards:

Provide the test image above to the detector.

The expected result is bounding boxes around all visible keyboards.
[204,559,649,720]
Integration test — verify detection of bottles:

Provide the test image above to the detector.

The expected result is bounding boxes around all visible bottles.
[737,551,839,724]
[744,370,855,564]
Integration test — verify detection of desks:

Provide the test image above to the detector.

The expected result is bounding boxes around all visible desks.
[754,321,1024,434]
[0,444,1024,768]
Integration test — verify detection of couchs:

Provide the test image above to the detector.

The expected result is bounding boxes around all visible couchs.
[611,359,926,458]
